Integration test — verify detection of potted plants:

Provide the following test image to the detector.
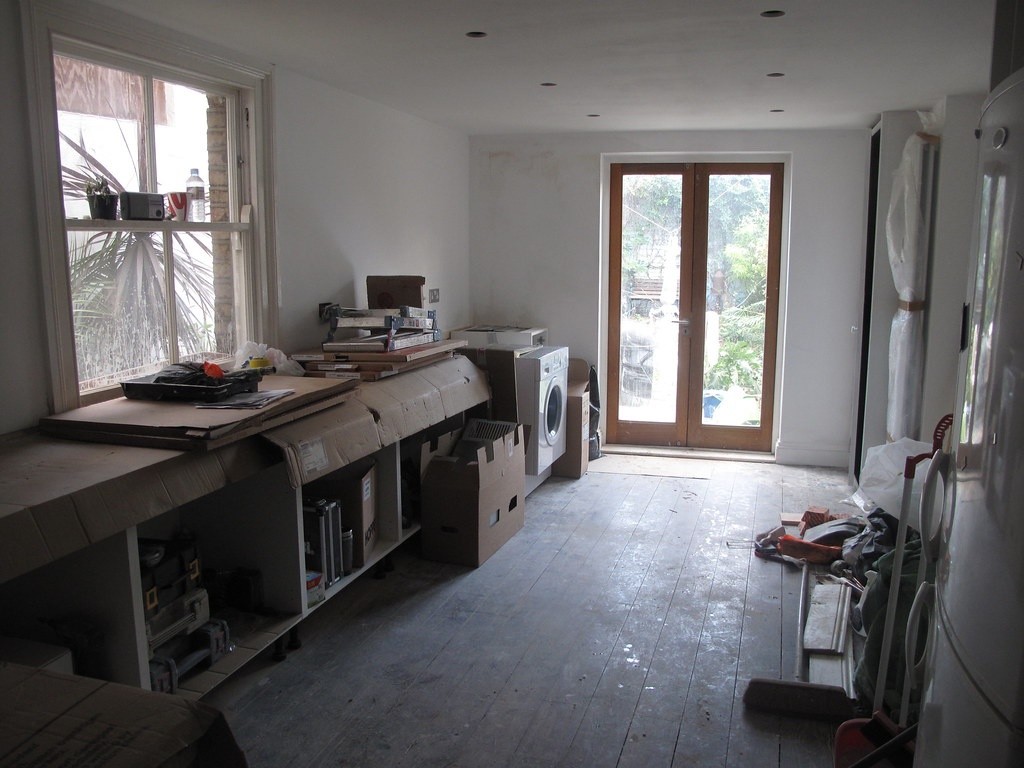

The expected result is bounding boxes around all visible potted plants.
[85,175,118,220]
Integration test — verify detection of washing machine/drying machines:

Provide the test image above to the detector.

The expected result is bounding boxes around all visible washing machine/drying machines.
[519,346,570,478]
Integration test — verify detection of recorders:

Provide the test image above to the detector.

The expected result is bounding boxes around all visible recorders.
[120,192,165,221]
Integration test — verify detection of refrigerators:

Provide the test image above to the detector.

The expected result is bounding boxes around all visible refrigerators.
[914,69,1024,768]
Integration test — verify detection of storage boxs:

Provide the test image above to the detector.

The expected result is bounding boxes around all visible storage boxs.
[0,659,249,768]
[551,358,590,478]
[308,459,379,590]
[418,418,525,568]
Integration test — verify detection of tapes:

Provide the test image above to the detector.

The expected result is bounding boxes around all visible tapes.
[249,357,270,369]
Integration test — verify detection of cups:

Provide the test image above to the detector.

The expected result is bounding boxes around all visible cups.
[167,192,193,222]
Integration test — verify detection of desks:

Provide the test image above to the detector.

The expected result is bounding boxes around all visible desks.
[0,353,490,698]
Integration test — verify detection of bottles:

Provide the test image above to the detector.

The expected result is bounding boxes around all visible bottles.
[186,168,206,222]
[341,527,353,576]
